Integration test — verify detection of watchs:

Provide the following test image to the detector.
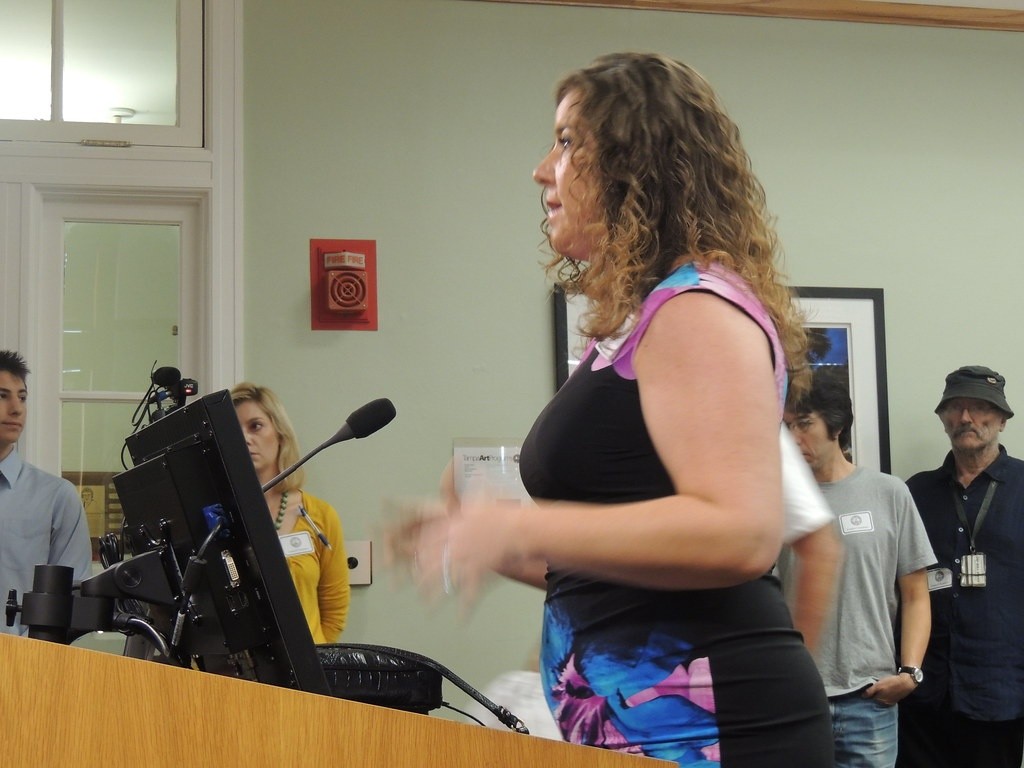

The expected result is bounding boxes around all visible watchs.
[896,666,924,687]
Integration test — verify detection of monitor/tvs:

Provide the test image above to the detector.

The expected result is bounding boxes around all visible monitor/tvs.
[113,388,333,698]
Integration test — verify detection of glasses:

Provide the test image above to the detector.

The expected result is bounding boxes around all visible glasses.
[787,415,822,432]
[946,403,997,418]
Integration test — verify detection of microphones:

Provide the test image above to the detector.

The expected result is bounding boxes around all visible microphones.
[262,398,397,493]
[152,366,181,387]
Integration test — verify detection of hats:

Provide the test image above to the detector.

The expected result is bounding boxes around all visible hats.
[935,366,1014,420]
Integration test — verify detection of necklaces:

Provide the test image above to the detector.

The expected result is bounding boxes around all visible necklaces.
[274,488,288,532]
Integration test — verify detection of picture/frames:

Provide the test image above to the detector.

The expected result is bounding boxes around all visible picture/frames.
[551,282,892,476]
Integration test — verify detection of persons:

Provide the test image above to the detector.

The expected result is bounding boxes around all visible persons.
[228,381,349,643]
[780,417,846,652]
[394,53,836,766]
[0,349,92,637]
[892,367,1024,767]
[781,368,940,767]
